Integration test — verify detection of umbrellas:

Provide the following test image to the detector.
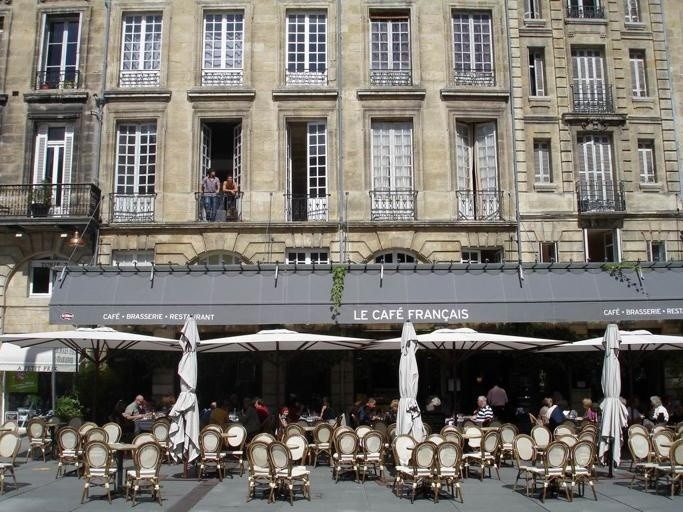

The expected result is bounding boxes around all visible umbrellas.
[395,319,428,443]
[599,324,629,479]
[359,327,572,426]
[531,329,683,419]
[168,314,200,478]
[0,326,180,422]
[197,328,377,409]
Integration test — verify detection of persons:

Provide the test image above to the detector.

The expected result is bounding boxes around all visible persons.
[200,168,220,222]
[222,174,238,221]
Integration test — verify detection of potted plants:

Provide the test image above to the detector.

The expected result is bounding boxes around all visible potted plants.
[23,178,52,217]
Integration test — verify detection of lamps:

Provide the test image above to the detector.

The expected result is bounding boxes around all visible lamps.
[65,226,87,247]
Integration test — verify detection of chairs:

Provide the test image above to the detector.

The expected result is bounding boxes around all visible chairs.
[197,419,388,504]
[55,422,169,506]
[1,415,62,491]
[389,416,598,506]
[627,419,683,499]
